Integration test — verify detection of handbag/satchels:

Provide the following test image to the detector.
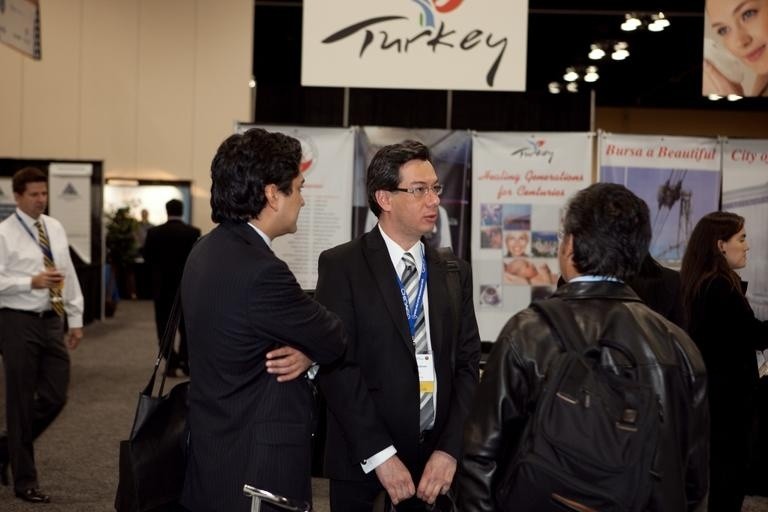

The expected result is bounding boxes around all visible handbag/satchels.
[118,375,189,512]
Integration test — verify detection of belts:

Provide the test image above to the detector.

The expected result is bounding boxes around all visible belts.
[18,309,59,320]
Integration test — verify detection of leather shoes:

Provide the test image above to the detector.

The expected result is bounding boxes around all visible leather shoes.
[16,488,49,502]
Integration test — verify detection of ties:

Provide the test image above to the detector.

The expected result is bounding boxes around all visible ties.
[35,221,64,317]
[401,253,434,434]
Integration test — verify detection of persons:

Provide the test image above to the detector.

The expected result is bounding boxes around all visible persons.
[555,249,684,320]
[453,180,708,509]
[1,167,87,500]
[135,209,157,248]
[704,1,767,99]
[314,142,480,509]
[178,124,346,512]
[146,199,199,375]
[505,230,529,255]
[104,207,137,300]
[483,205,501,224]
[501,256,562,285]
[682,210,766,509]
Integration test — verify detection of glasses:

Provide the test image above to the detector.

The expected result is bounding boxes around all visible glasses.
[393,184,443,198]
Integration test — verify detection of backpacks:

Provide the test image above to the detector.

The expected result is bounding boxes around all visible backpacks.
[498,299,661,512]
